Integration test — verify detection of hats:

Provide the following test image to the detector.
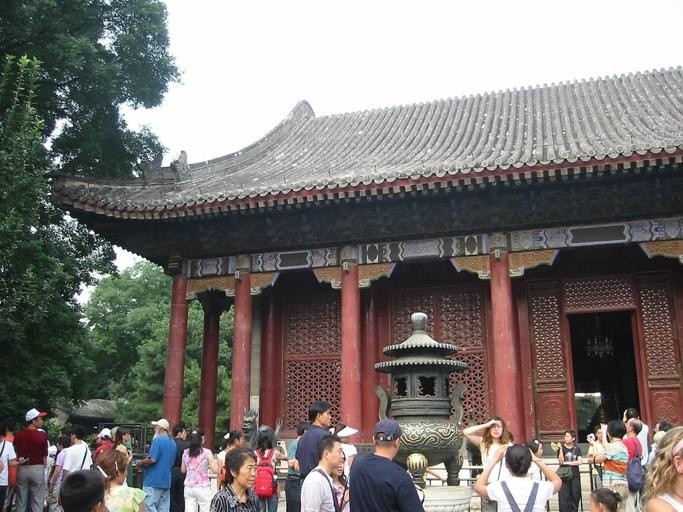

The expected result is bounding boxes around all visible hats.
[151,418,170,430]
[374,419,402,441]
[100,428,112,438]
[337,426,358,437]
[25,408,48,422]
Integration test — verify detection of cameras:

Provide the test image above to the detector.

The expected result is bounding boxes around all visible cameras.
[587,433,596,441]
[277,441,282,446]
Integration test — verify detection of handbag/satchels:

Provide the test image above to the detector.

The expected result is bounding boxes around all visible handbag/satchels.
[557,467,573,482]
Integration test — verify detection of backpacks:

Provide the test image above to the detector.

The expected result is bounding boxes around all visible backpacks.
[628,457,644,491]
[253,449,278,498]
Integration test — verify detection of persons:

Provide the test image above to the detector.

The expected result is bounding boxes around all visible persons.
[1,408,288,512]
[285,402,424,511]
[463,408,683,512]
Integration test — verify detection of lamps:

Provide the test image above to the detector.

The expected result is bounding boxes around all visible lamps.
[585,336,615,361]
